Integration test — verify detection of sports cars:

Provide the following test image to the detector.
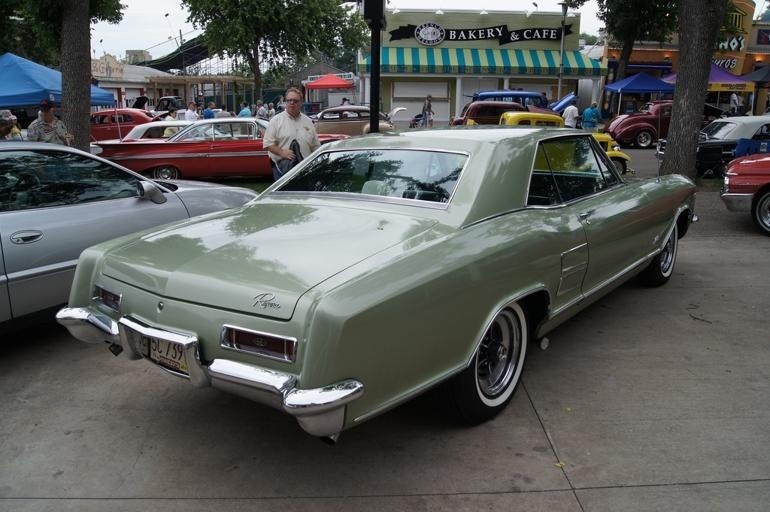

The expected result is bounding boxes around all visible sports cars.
[55,125,698,446]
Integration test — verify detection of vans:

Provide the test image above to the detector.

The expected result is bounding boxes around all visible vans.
[463,90,576,114]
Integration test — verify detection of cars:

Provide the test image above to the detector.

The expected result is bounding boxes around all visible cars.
[90,109,171,143]
[90,121,232,162]
[734,137,770,158]
[467,111,636,176]
[308,101,407,139]
[603,100,732,150]
[654,116,770,180]
[448,101,561,127]
[276,102,287,110]
[1,140,260,324]
[150,95,188,116]
[720,153,770,237]
[92,117,352,180]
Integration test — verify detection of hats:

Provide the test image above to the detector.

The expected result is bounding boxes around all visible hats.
[36,100,54,109]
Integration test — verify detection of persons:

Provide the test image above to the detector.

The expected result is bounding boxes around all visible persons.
[562,100,579,128]
[0,108,23,140]
[581,102,602,130]
[261,88,321,184]
[422,94,436,128]
[165,95,353,137]
[738,92,745,112]
[729,91,737,114]
[26,101,69,146]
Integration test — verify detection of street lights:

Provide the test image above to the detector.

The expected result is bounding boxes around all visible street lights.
[557,0,573,102]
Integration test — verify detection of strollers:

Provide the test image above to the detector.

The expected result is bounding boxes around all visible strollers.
[408,114,424,129]
